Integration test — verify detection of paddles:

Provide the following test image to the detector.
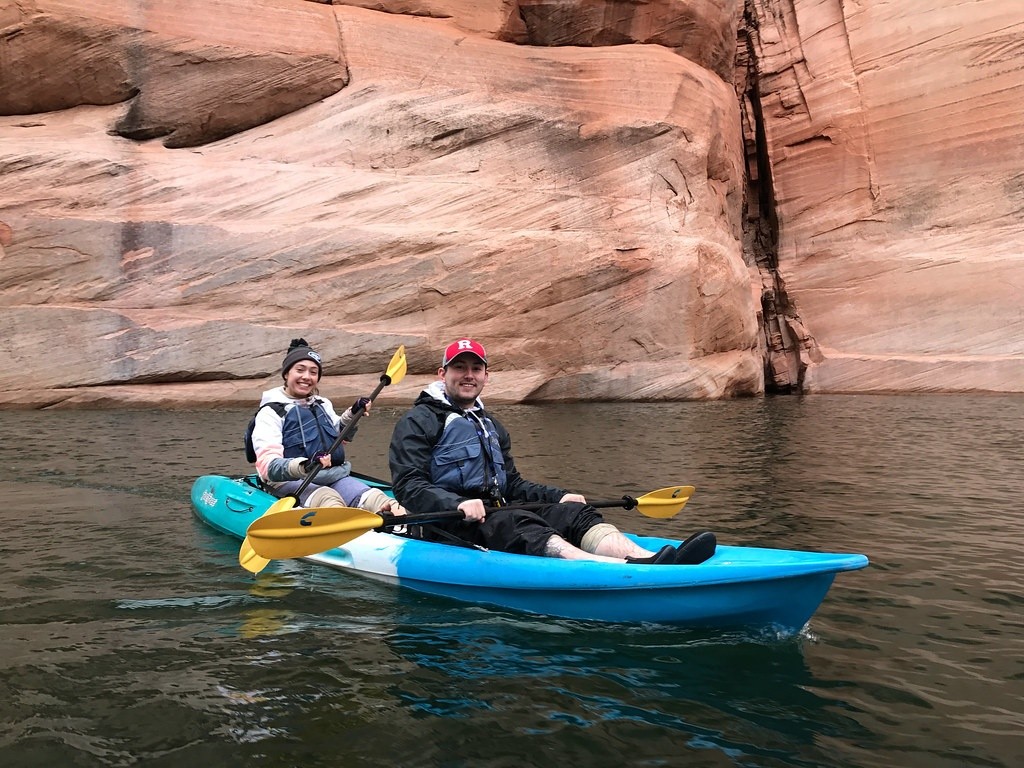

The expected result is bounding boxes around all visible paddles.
[246,485,695,560]
[238,345,407,573]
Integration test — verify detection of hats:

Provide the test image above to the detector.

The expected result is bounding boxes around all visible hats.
[282,337,322,383]
[442,339,487,368]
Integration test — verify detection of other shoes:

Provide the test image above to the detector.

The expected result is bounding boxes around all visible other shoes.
[625,545,676,564]
[676,530,716,565]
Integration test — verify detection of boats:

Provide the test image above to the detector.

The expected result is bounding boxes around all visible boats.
[190,470,870,639]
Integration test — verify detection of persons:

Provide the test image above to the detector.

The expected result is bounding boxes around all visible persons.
[251,338,407,534]
[389,338,716,565]
